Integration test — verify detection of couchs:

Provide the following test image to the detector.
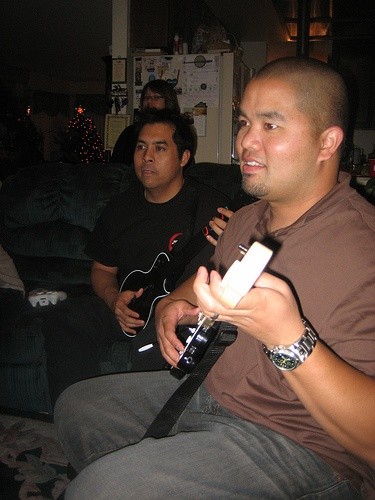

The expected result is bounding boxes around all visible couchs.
[0,161,244,417]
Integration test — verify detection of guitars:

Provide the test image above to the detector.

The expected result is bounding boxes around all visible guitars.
[117,205,235,338]
[169,240,274,379]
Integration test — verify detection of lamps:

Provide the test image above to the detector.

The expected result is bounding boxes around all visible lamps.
[283,0,330,44]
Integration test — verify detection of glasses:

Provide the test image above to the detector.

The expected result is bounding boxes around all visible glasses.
[141,93,164,101]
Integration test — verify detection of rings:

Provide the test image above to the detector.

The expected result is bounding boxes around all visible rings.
[211,314,219,319]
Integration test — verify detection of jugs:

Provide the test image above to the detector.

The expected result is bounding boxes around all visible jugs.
[350,145,366,165]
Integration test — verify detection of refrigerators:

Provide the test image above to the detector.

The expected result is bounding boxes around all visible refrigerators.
[135,53,252,165]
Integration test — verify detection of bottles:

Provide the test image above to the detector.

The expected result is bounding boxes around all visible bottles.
[368,144,375,178]
[173,34,189,55]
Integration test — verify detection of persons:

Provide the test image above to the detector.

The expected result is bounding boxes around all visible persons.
[53,55,375,500]
[0,244,27,313]
[91,110,235,372]
[113,78,195,164]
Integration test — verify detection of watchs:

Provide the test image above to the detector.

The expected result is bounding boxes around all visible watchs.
[262,318,318,370]
